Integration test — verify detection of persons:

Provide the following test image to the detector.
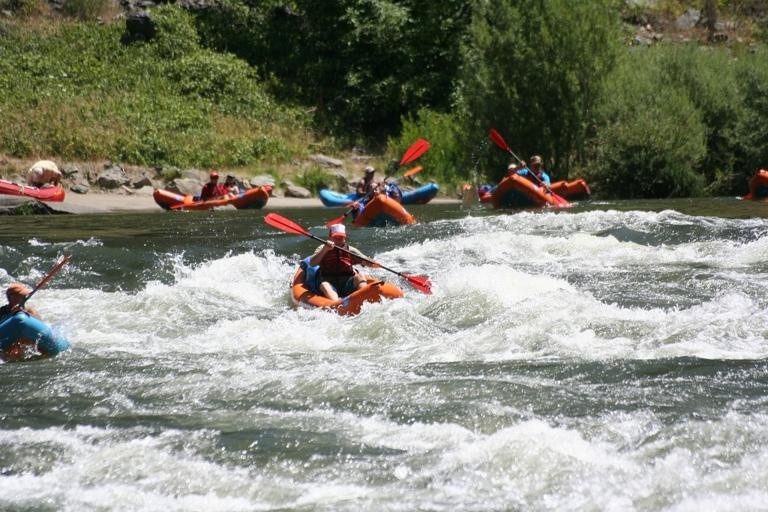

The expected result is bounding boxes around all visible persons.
[357,166,376,198]
[351,182,388,223]
[508,155,550,194]
[201,172,223,202]
[223,174,240,198]
[310,223,381,302]
[0,284,41,328]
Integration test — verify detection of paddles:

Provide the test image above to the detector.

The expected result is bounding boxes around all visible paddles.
[325,138,430,228]
[491,129,571,208]
[264,213,433,295]
[0,255,73,321]
[345,165,424,207]
[170,185,273,210]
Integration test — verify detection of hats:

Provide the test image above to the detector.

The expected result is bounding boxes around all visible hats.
[6,286,30,297]
[365,166,375,173]
[530,156,541,165]
[210,172,218,178]
[228,172,235,179]
[329,224,346,239]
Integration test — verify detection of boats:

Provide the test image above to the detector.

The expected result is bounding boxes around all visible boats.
[263,185,272,194]
[290,265,404,317]
[320,183,440,207]
[0,180,66,202]
[352,194,416,227]
[0,309,69,356]
[748,169,768,198]
[491,175,559,207]
[153,184,269,211]
[478,178,591,202]
